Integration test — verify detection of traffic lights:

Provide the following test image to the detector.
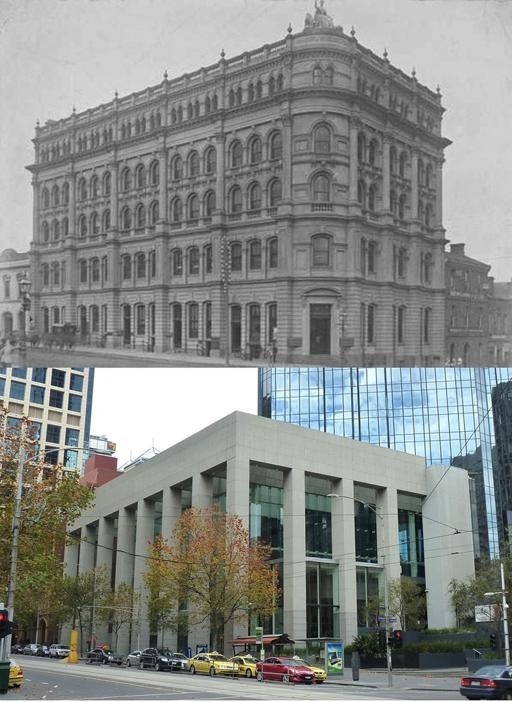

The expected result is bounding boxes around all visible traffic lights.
[0,610,16,638]
[489,633,498,651]
[390,629,402,649]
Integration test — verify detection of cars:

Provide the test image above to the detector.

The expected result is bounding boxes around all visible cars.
[460,664,512,699]
[87,648,326,685]
[12,643,69,658]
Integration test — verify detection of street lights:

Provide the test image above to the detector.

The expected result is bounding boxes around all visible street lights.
[4,446,116,660]
[326,493,392,688]
[484,562,510,666]
